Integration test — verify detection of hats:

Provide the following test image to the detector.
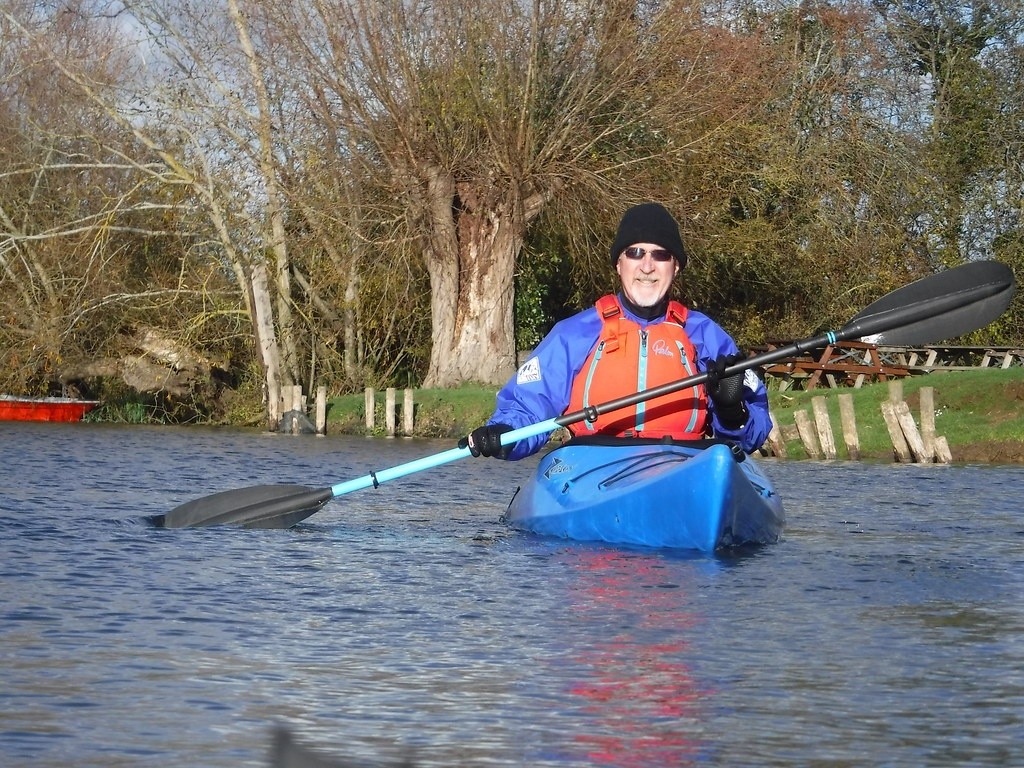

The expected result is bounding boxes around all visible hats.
[609,203,687,272]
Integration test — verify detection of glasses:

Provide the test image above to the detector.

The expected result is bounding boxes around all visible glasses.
[622,247,680,261]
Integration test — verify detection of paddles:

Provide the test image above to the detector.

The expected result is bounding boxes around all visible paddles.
[157,258,1018,529]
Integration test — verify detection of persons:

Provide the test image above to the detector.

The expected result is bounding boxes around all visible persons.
[457,202,774,461]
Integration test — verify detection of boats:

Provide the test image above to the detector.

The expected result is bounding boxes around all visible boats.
[500,435,784,557]
[0,395,105,423]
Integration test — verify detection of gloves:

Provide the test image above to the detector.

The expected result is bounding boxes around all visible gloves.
[458,423,517,459]
[705,350,749,430]
